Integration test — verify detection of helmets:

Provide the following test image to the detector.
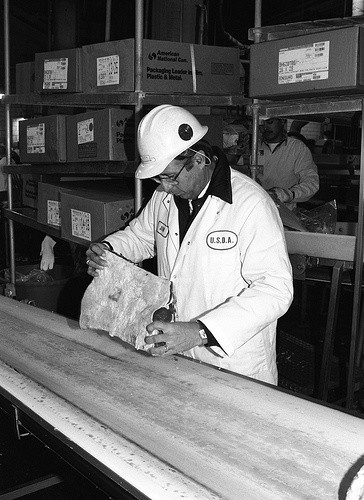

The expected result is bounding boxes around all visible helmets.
[134,104,209,179]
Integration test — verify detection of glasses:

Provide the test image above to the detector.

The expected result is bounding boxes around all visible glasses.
[150,154,196,186]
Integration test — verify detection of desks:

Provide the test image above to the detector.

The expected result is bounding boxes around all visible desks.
[0,296,364,500]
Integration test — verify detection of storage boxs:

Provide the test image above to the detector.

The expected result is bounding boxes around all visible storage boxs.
[13,25,364,253]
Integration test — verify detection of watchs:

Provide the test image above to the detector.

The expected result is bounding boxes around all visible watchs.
[197,321,207,346]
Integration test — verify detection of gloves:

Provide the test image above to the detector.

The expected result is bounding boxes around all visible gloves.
[270,187,294,203]
[39,235,57,270]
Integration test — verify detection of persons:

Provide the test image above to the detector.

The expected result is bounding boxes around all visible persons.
[86,103,294,386]
[0,144,75,273]
[250,117,319,212]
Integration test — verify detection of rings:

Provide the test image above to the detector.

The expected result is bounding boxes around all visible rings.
[86,260,91,265]
[165,346,168,352]
